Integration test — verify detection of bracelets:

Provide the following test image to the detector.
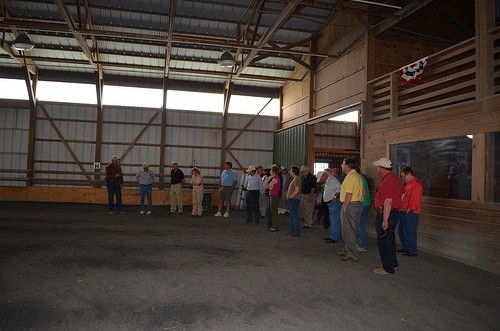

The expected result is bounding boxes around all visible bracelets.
[311,195,313,196]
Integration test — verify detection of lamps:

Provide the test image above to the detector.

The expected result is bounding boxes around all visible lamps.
[12,32,35,51]
[217,50,236,68]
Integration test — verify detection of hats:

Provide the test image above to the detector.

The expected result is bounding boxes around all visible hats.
[143,162,149,167]
[246,165,256,172]
[373,157,392,168]
[112,156,120,160]
[300,164,310,171]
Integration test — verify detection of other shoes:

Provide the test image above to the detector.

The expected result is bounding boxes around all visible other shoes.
[337,251,359,263]
[223,212,230,217]
[302,226,310,228]
[373,266,391,275]
[397,249,417,256]
[189,214,196,217]
[324,237,340,243]
[285,231,299,237]
[116,211,125,215]
[357,247,368,251]
[264,223,272,228]
[109,211,114,214]
[244,221,252,224]
[147,210,152,215]
[139,210,145,215]
[168,212,177,214]
[178,212,182,215]
[267,228,280,232]
[253,222,260,225]
[214,211,222,216]
[198,215,203,217]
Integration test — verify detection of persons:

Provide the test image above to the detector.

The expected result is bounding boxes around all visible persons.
[105,156,124,214]
[190,168,204,217]
[135,163,153,214]
[316,167,346,243]
[244,164,290,232]
[286,166,302,238]
[300,166,318,229]
[337,156,365,262]
[396,166,423,257]
[357,169,371,253]
[169,162,185,215]
[214,161,238,217]
[373,159,405,276]
